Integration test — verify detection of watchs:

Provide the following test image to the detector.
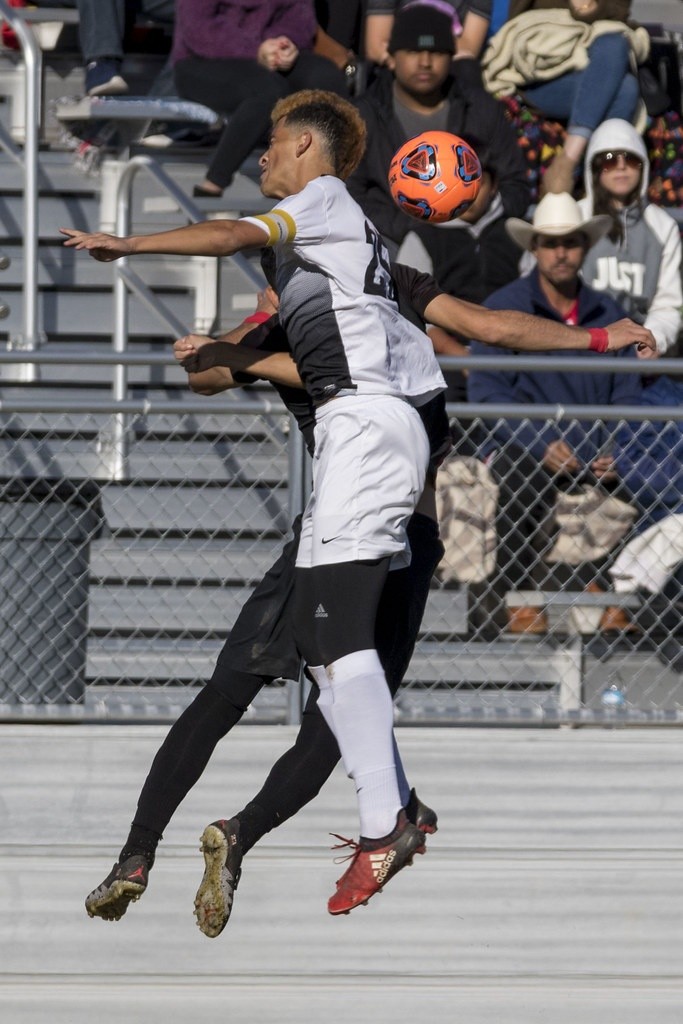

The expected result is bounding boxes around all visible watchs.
[343,62,356,76]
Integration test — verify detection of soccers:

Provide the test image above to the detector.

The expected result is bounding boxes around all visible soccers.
[388,129,486,223]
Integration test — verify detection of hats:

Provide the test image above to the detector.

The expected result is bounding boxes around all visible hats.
[387,2,458,58]
[505,192,613,253]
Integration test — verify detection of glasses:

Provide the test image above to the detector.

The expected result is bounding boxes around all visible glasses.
[596,150,643,170]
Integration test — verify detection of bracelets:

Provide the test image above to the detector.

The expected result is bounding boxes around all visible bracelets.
[246,312,271,324]
[588,327,608,352]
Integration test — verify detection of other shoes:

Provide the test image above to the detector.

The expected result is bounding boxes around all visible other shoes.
[187,185,223,226]
[594,627,646,651]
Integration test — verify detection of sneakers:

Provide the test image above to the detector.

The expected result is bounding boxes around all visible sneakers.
[85,57,129,97]
[539,147,580,203]
[327,787,439,915]
[193,817,244,938]
[85,855,149,921]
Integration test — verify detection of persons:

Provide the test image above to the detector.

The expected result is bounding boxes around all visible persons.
[57,87,438,916]
[83,261,655,937]
[0,0,683,669]
